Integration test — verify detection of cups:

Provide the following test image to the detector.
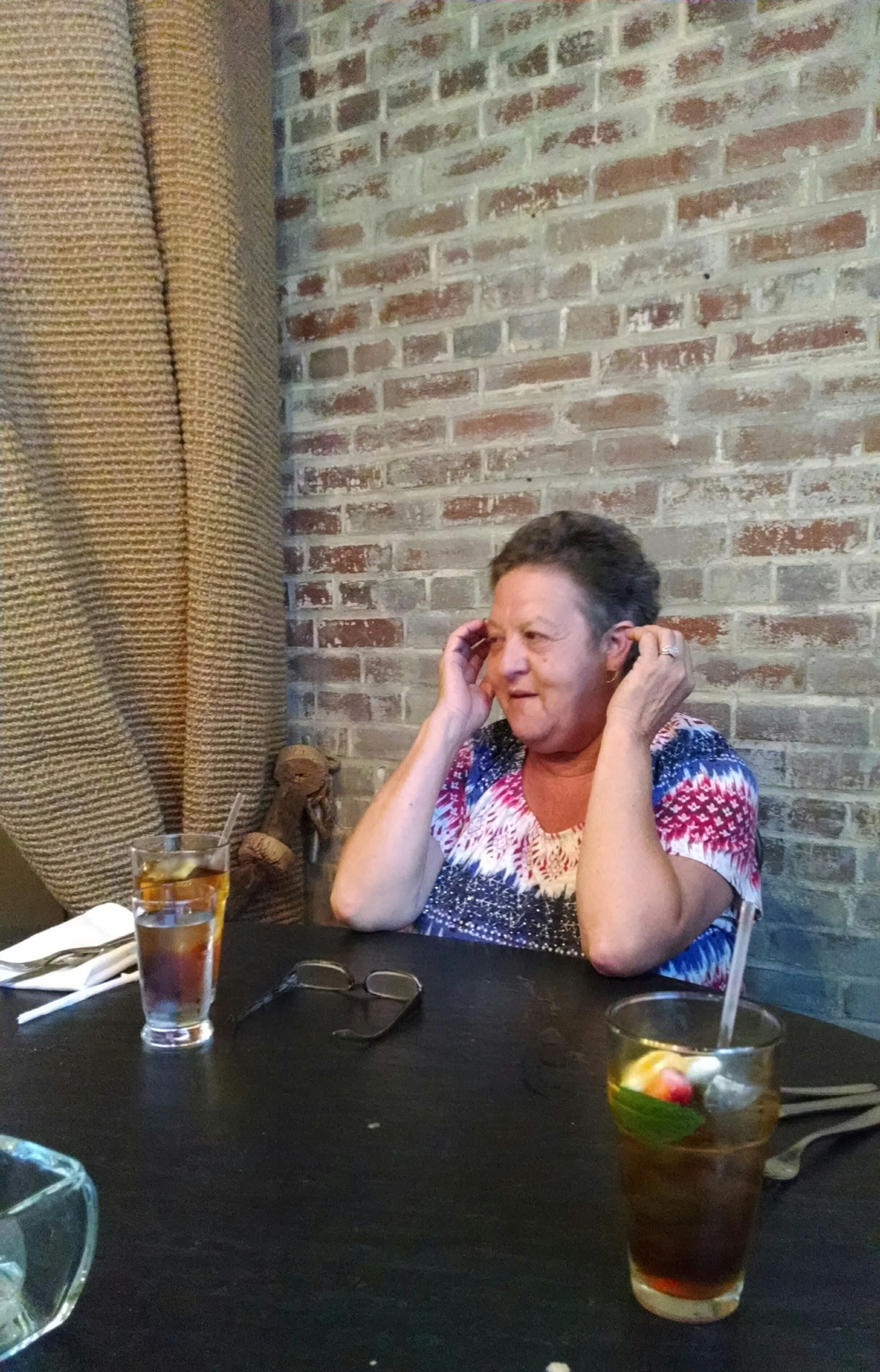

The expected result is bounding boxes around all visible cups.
[605,991,786,1325]
[129,834,229,1049]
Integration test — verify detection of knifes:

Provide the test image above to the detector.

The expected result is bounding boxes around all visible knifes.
[0,937,135,987]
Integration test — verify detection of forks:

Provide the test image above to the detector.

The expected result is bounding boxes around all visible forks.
[764,1103,880,1179]
[0,931,135,973]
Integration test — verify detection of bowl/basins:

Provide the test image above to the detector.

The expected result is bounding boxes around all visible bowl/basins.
[0,1134,99,1363]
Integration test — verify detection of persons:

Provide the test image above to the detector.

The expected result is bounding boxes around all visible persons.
[331,513,761,998]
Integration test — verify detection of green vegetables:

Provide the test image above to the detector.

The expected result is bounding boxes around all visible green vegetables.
[612,1087,705,1143]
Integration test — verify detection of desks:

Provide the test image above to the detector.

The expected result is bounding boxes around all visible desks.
[0,922,880,1372]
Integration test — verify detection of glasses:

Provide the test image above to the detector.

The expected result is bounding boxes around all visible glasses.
[227,960,425,1042]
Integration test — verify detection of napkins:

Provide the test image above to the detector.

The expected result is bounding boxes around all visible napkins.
[0,903,137,991]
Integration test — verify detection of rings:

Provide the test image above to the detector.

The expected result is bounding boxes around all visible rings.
[658,645,681,659]
